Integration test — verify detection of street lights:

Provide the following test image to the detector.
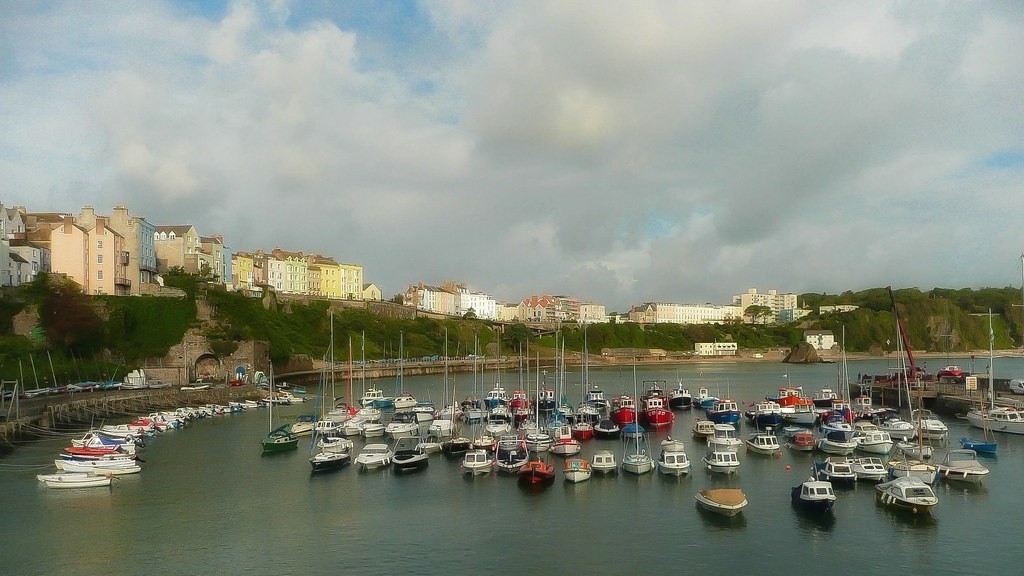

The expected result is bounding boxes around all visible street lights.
[985,365,990,402]
[923,361,928,391]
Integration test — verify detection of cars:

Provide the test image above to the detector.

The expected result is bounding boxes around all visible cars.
[1009,380,1024,395]
[0,378,213,399]
[257,383,269,390]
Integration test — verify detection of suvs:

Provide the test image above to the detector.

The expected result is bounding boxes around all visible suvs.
[936,366,973,383]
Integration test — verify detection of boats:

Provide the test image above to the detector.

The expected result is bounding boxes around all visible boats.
[692,421,716,438]
[669,370,692,410]
[909,409,949,440]
[855,384,873,413]
[765,365,813,407]
[706,400,743,424]
[291,412,319,435]
[290,383,306,395]
[692,385,720,409]
[855,410,877,421]
[874,476,939,515]
[694,488,749,518]
[877,407,902,423]
[781,404,817,425]
[706,424,744,454]
[812,456,856,490]
[850,457,889,483]
[787,434,816,451]
[895,436,934,462]
[657,436,691,479]
[783,425,814,438]
[744,391,787,426]
[886,460,940,485]
[37,393,306,489]
[816,416,858,456]
[701,451,740,474]
[851,422,894,454]
[934,450,989,484]
[789,480,837,513]
[746,427,780,455]
[611,393,638,427]
[812,385,844,416]
[639,378,675,432]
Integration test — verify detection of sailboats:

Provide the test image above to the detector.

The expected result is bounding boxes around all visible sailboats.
[830,324,855,423]
[966,308,1024,435]
[960,390,997,454]
[260,361,299,452]
[620,358,656,475]
[307,312,620,487]
[878,318,916,440]
